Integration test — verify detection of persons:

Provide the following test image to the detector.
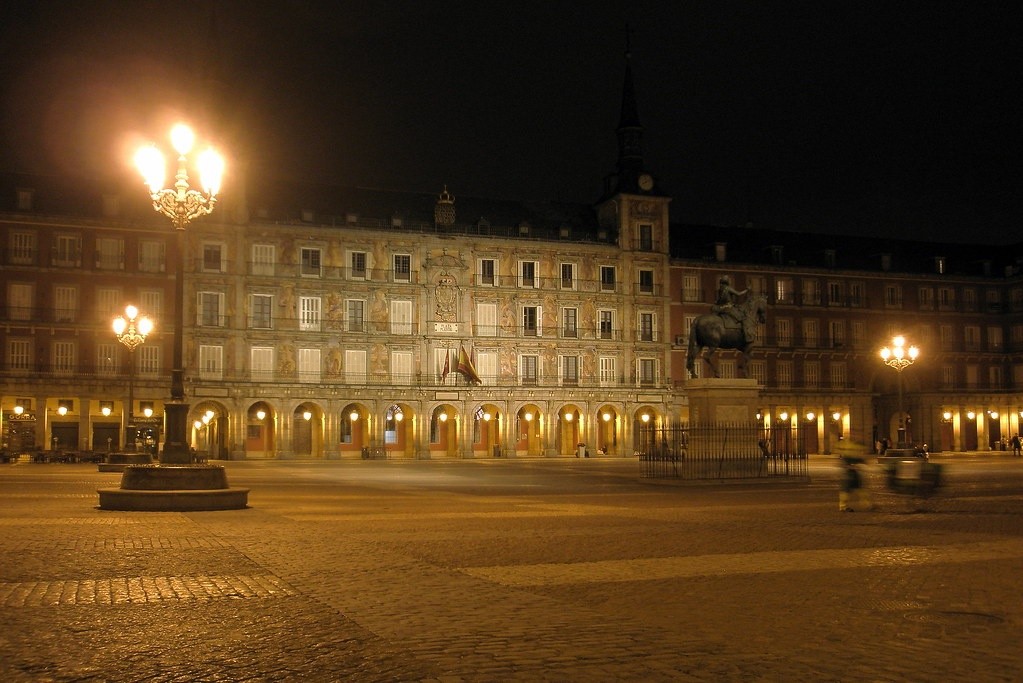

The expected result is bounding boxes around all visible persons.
[876,438,893,458]
[1000,433,1023,456]
[710,275,755,343]
[913,442,929,461]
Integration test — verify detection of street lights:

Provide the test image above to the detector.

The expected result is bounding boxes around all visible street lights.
[112,305,153,454]
[879,335,920,450]
[133,119,227,466]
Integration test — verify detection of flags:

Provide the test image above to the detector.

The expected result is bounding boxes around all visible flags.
[458,344,482,385]
[442,350,449,383]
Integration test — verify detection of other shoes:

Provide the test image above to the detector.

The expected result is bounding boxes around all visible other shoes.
[844,507,854,512]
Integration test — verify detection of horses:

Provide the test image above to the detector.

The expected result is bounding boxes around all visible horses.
[686,292,769,378]
